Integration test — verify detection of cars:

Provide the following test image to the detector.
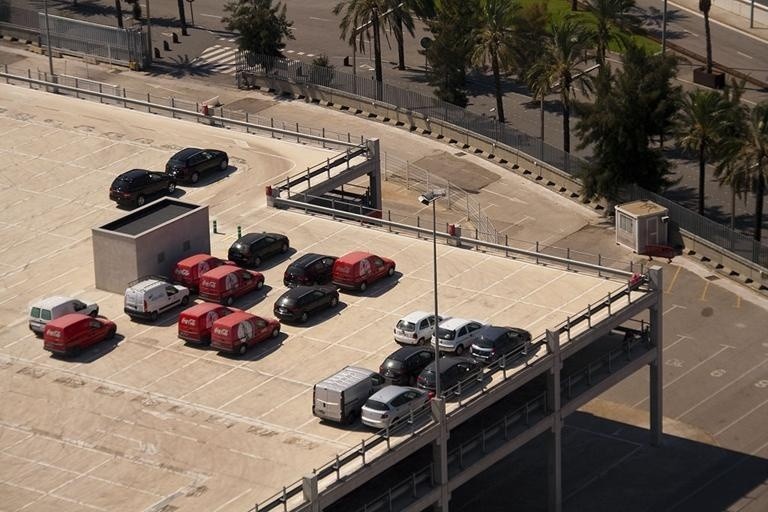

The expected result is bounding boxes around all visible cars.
[417,355,484,396]
[380,346,443,385]
[283,254,339,288]
[109,168,176,207]
[431,317,493,355]
[470,327,533,367]
[274,286,339,324]
[360,384,435,429]
[227,232,291,269]
[391,311,453,346]
[166,148,228,185]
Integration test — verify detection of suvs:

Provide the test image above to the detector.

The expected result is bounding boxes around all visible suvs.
[124,274,190,322]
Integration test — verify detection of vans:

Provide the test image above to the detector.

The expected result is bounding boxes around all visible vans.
[43,314,118,358]
[27,297,100,336]
[176,302,243,346]
[334,251,396,292]
[311,364,387,425]
[212,311,281,357]
[173,253,265,306]
